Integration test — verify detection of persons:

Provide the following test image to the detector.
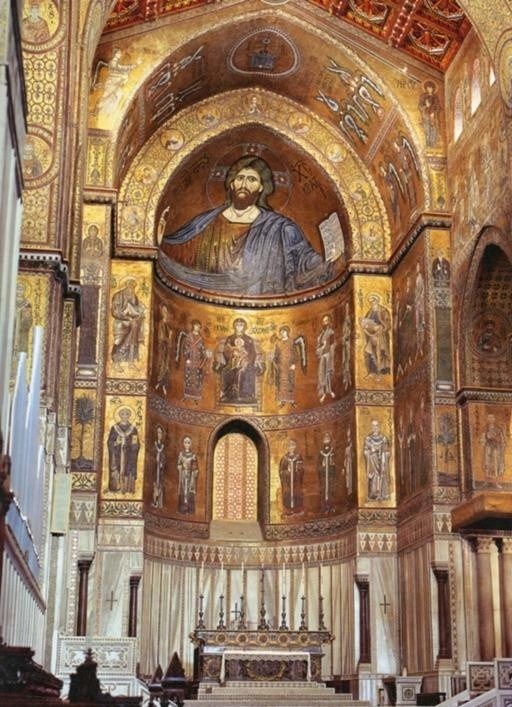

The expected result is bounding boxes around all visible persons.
[361,417,391,500]
[478,411,506,491]
[314,312,337,404]
[341,426,355,500]
[277,436,307,519]
[228,338,249,368]
[154,303,172,395]
[79,225,101,274]
[178,317,208,399]
[431,248,450,286]
[339,302,352,392]
[150,425,168,509]
[215,313,261,399]
[358,291,392,384]
[108,274,143,367]
[392,257,430,499]
[174,434,200,513]
[268,325,300,411]
[158,153,328,297]
[104,406,139,497]
[315,430,338,513]
[415,79,441,144]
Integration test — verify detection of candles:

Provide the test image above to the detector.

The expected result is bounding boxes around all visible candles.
[197,560,326,597]
